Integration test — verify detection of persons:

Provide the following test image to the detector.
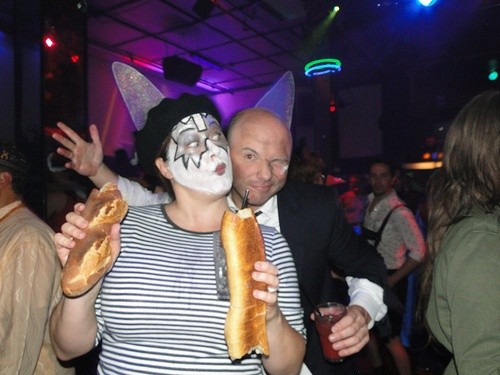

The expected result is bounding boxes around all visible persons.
[287,148,434,225]
[52,107,388,375]
[49,92,306,375]
[361,159,426,375]
[0,138,76,375]
[420,91,500,375]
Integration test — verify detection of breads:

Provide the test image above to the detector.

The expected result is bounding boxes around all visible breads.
[221,207,271,361]
[59,180,128,296]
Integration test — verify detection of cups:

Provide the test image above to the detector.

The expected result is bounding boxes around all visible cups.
[314,303,347,362]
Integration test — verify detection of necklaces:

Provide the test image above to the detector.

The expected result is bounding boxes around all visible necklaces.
[0,203,25,223]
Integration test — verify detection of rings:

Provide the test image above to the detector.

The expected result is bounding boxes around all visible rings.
[268,286,279,292]
